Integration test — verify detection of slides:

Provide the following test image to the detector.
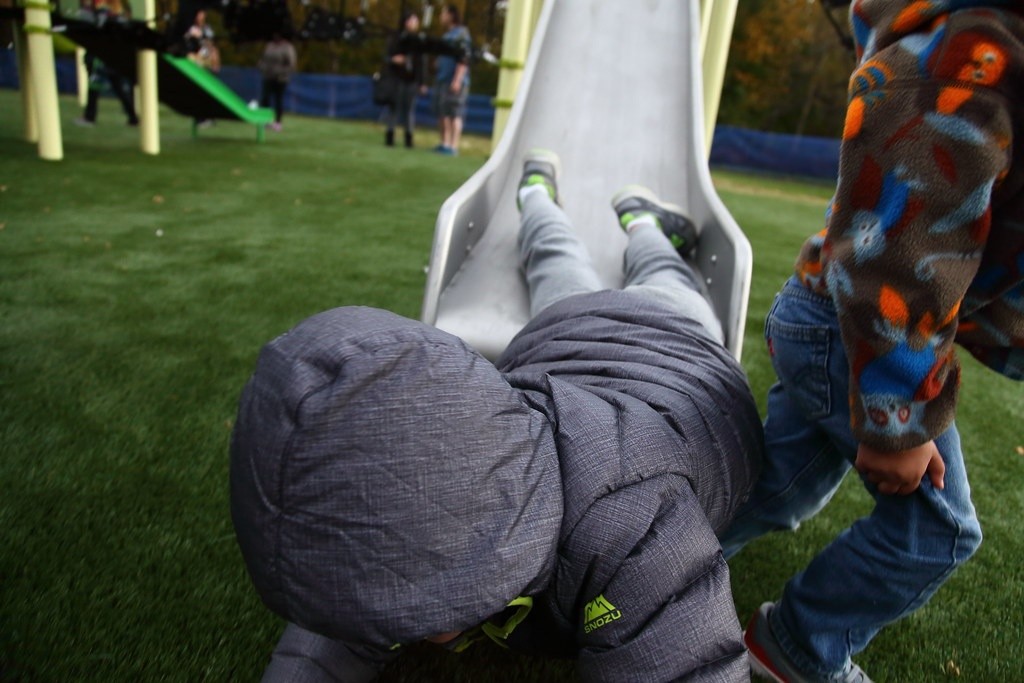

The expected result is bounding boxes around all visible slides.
[60,4,276,125]
[419,1,757,366]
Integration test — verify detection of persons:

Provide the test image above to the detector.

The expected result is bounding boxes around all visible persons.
[258,38,296,131]
[384,9,428,147]
[230,147,770,683]
[185,11,213,67]
[431,5,471,154]
[70,0,139,127]
[738,0,1024,682]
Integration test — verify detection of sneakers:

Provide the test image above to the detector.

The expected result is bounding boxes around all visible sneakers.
[606,183,699,258]
[742,601,873,683]
[515,149,560,216]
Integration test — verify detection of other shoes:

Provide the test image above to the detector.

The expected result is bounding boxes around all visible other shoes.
[263,121,281,131]
[432,143,459,157]
[385,130,394,144]
[404,134,415,148]
